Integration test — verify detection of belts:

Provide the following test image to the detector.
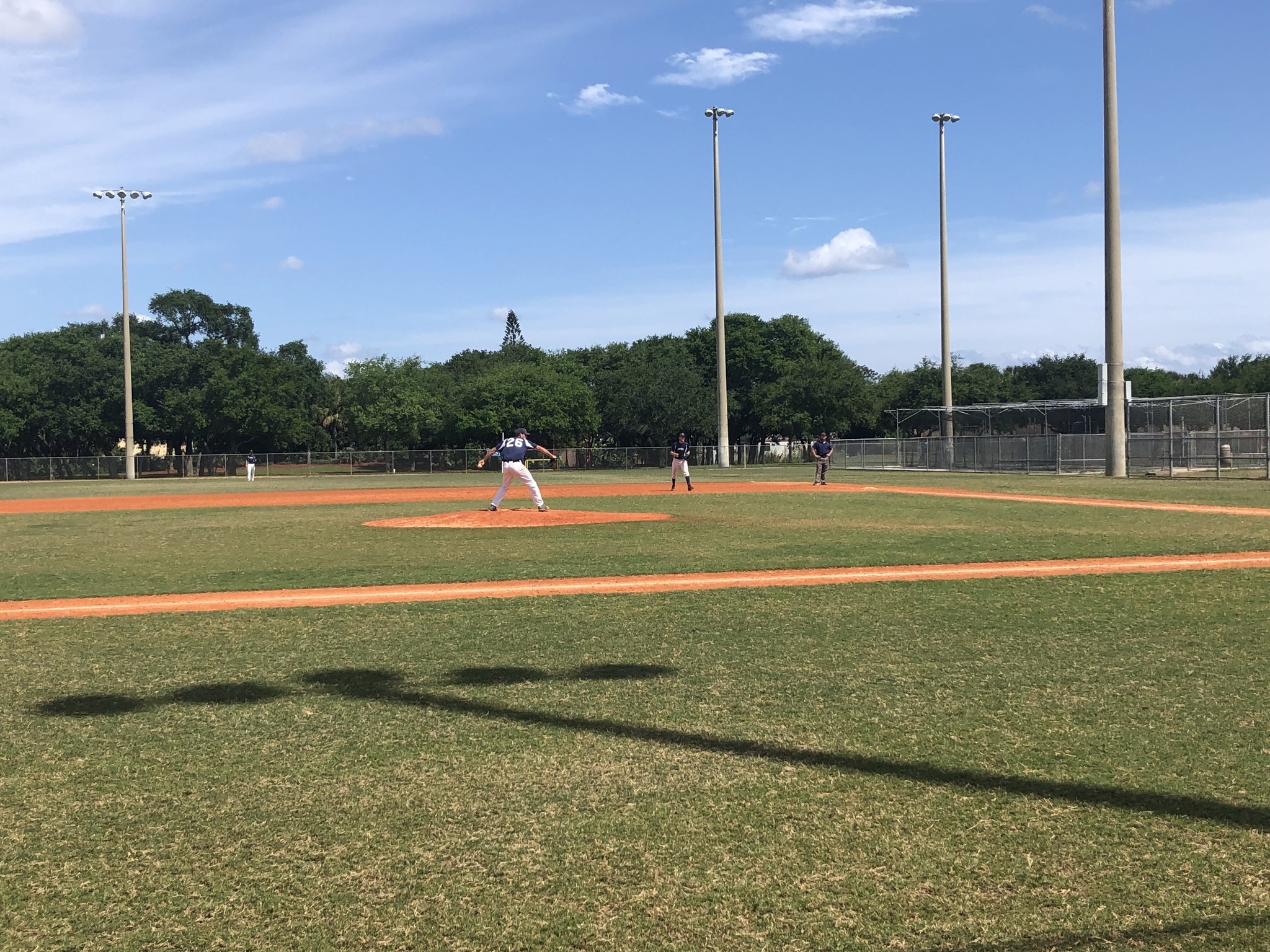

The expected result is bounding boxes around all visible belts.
[507,460,519,462]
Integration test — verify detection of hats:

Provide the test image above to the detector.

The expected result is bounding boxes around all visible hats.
[679,433,685,436]
[821,432,826,435]
[515,428,531,436]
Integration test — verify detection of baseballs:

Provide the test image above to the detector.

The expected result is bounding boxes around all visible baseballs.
[478,464,482,467]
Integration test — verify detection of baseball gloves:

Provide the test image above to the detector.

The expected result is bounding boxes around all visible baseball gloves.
[549,456,557,467]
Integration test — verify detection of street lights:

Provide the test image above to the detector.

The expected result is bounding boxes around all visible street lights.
[704,105,735,470]
[92,186,152,481]
[931,112,961,470]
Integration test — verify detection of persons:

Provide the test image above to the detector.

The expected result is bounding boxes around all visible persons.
[477,428,557,511]
[669,433,694,492]
[811,432,834,486]
[246,450,257,482]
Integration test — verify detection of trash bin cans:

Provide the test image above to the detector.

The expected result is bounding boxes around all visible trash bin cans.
[1221,444,1231,466]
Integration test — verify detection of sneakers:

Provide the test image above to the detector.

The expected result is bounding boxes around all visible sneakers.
[538,504,549,511]
[487,505,497,511]
[670,485,675,491]
[813,482,819,485]
[821,483,827,485]
[688,485,694,491]
